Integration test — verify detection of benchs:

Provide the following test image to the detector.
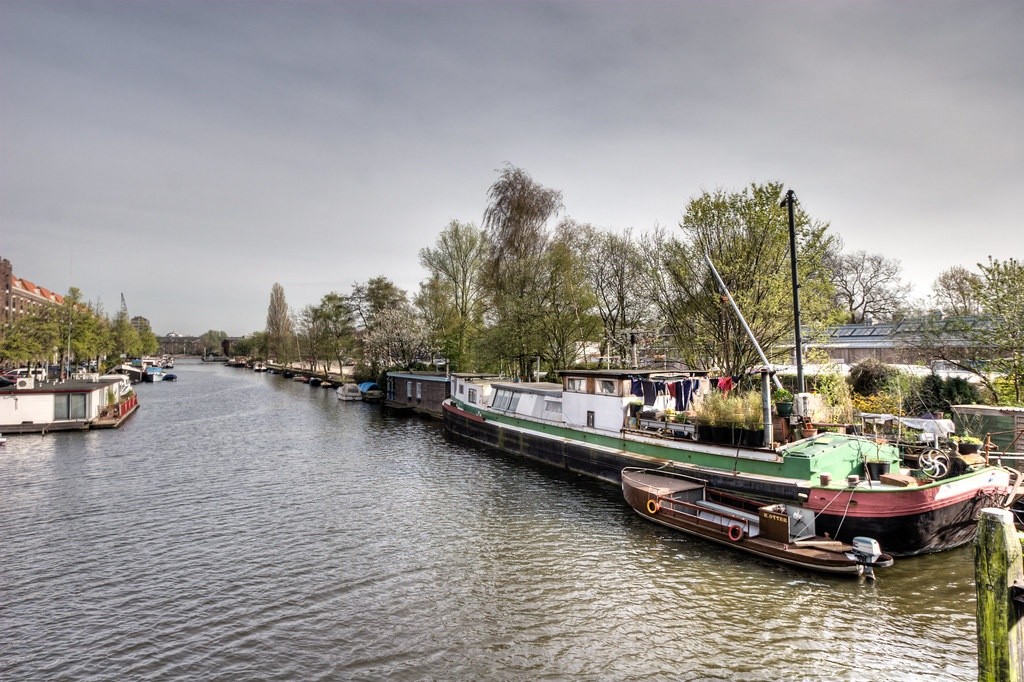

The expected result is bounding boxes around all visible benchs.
[696,499,759,537]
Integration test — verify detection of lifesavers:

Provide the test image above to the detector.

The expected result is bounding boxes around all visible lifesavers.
[728,525,745,541]
[646,498,660,514]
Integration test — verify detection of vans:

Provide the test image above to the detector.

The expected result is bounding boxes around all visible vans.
[5,369,45,381]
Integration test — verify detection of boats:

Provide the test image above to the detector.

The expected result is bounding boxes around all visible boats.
[359,382,385,400]
[115,360,147,384]
[440,189,1011,557]
[336,383,361,401]
[143,367,166,382]
[621,465,895,579]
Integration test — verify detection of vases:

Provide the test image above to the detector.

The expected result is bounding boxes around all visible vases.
[802,428,818,437]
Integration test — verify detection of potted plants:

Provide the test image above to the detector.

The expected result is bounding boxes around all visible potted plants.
[774,388,794,417]
[630,402,643,417]
[693,387,764,447]
[946,436,983,454]
